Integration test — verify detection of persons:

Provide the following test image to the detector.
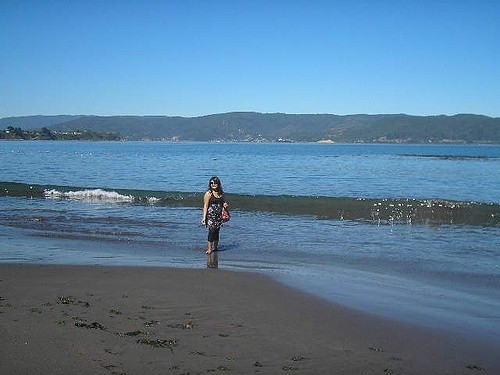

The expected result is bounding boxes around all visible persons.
[201,176,228,254]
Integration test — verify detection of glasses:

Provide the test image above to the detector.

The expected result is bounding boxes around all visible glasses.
[210,182,217,184]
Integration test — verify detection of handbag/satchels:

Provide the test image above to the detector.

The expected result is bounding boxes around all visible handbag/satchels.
[222,206,230,222]
